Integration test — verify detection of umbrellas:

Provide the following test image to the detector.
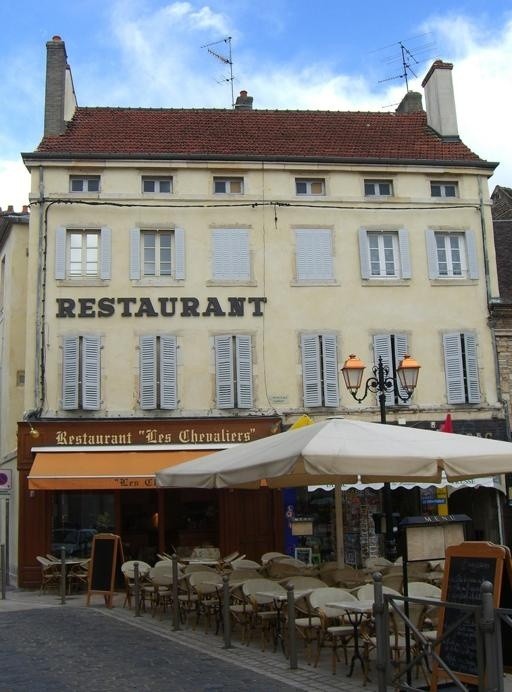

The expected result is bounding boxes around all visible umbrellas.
[157,417,512,569]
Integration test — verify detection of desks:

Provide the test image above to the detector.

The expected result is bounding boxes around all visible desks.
[325,599,409,680]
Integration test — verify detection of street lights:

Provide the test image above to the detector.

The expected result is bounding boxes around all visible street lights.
[340,353,422,563]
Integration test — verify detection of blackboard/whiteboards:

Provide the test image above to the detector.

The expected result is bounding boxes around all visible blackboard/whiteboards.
[87,533,118,594]
[499,545,512,673]
[433,542,506,687]
[114,536,129,592]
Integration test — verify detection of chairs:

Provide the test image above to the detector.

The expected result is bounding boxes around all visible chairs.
[222,552,246,564]
[309,588,374,675]
[156,553,175,562]
[178,563,262,636]
[36,554,91,595]
[261,552,440,585]
[361,608,417,690]
[356,585,404,610]
[402,582,440,601]
[242,576,329,660]
[421,608,440,641]
[120,559,177,621]
